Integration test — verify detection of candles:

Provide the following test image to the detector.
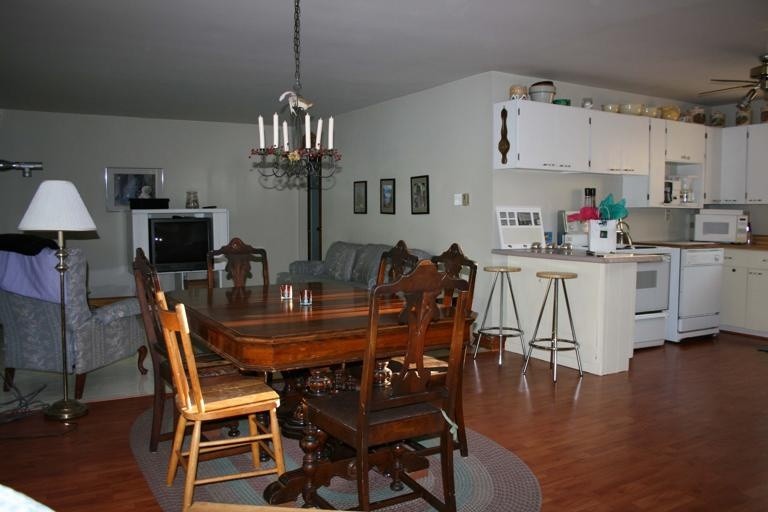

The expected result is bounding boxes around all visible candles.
[283,120,288,152]
[258,116,264,150]
[305,113,311,148]
[315,118,322,148]
[328,116,334,149]
[273,112,279,148]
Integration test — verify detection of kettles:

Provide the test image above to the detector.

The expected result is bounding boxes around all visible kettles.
[616,220,632,249]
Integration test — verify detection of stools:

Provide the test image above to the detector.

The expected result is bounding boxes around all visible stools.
[471,266,526,365]
[522,272,587,383]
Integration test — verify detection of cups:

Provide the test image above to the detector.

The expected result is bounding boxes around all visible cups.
[299,288,313,306]
[280,283,293,302]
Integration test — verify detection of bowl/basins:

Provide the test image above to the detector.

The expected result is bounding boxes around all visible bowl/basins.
[530,85,555,103]
[622,103,646,116]
[642,105,662,118]
[602,104,620,112]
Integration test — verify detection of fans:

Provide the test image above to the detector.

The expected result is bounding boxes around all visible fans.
[697,56,767,112]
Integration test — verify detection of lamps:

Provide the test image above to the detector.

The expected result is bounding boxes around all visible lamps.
[18,179,98,421]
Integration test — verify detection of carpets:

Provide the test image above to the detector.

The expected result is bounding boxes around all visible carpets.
[128,396,544,511]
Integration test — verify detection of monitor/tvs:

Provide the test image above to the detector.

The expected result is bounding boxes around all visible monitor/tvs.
[149,218,214,272]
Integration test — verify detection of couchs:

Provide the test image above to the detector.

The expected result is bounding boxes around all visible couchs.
[276,241,433,290]
[0,233,149,399]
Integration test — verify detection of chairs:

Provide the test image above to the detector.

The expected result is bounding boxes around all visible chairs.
[156,290,285,511]
[378,241,416,286]
[208,237,269,288]
[129,244,247,454]
[303,260,478,508]
[391,242,478,459]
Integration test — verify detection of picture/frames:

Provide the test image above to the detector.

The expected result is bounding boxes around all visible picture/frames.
[380,178,395,214]
[409,175,429,214]
[104,167,165,212]
[353,181,366,214]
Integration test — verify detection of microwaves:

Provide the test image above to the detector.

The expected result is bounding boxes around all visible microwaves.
[694,213,748,245]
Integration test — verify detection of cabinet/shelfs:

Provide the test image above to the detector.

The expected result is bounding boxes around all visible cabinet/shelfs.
[747,124,768,204]
[494,101,591,172]
[720,242,767,340]
[591,109,651,177]
[623,116,705,210]
[703,125,748,207]
